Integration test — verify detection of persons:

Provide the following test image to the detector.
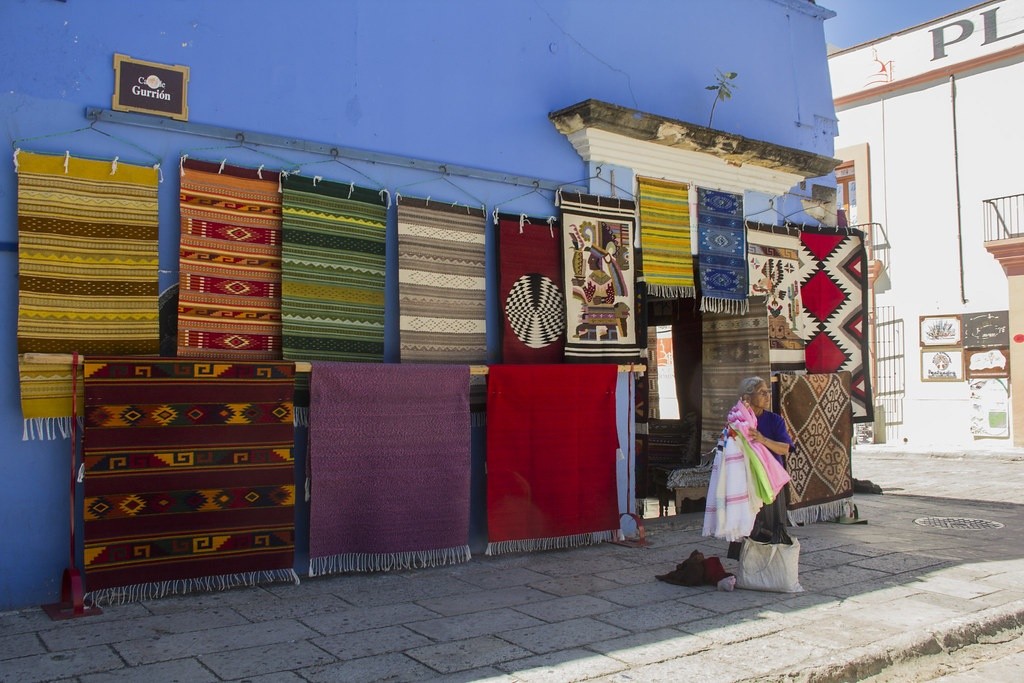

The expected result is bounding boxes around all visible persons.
[728,377,795,562]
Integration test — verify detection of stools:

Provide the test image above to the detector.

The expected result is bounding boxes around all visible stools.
[651,481,710,517]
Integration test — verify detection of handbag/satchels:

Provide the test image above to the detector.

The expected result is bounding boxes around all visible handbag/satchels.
[734,535,804,593]
